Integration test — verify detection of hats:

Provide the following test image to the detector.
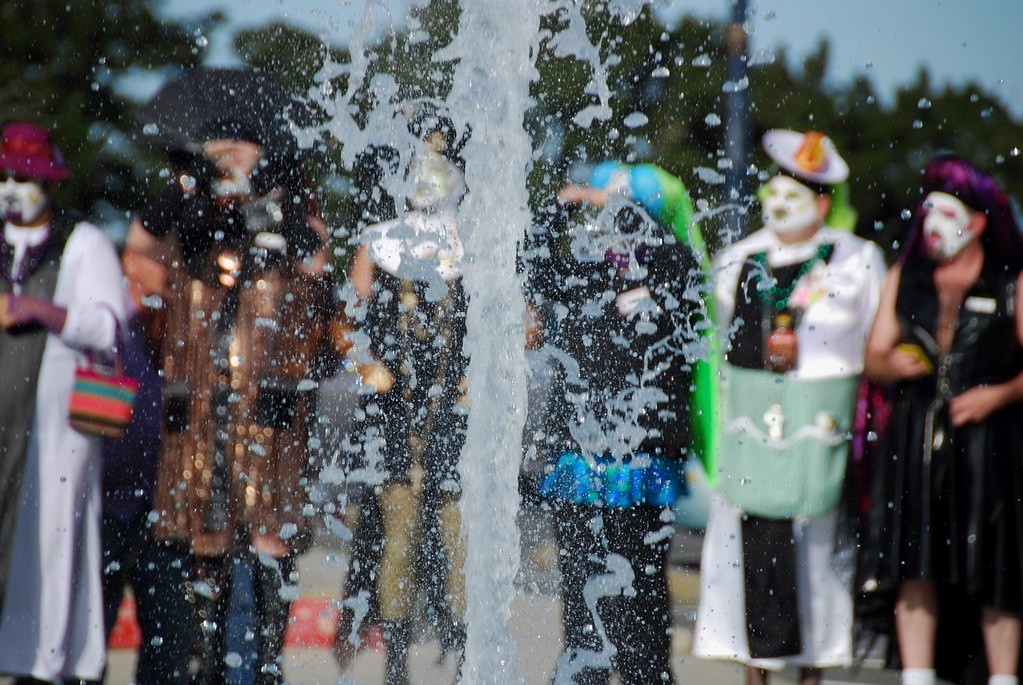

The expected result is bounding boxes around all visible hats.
[765,128,851,184]
[924,158,1008,217]
[0,122,71,179]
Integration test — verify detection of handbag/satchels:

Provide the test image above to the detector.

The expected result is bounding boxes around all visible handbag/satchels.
[69,304,140,438]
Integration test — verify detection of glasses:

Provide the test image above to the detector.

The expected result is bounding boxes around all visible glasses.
[0,171,31,184]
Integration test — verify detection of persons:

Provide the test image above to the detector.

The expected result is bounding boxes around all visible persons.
[1,121,465,685]
[519,115,1023,684]
